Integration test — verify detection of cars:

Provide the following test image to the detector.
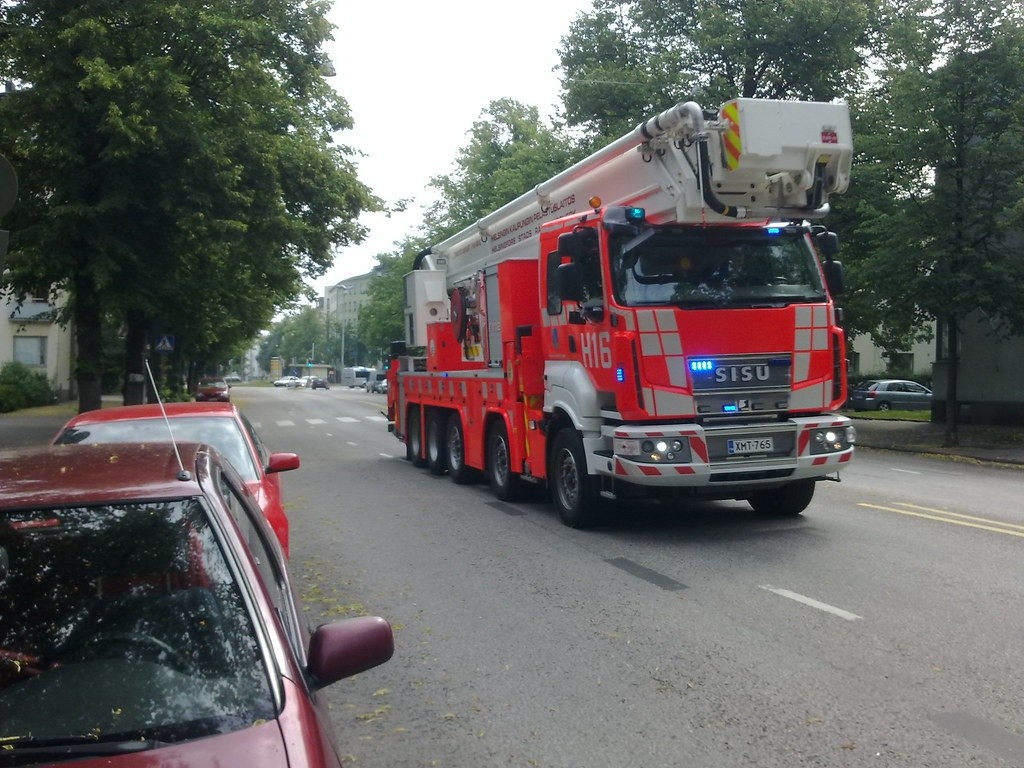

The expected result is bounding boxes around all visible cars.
[300,375,318,387]
[367,379,388,394]
[273,375,301,388]
[10,402,301,592]
[193,376,231,402]
[311,378,331,390]
[1,438,396,768]
[849,380,934,412]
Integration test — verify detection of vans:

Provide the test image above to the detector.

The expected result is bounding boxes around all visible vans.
[342,366,378,388]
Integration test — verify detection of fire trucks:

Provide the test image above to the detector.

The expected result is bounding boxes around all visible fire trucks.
[379,98,856,530]
